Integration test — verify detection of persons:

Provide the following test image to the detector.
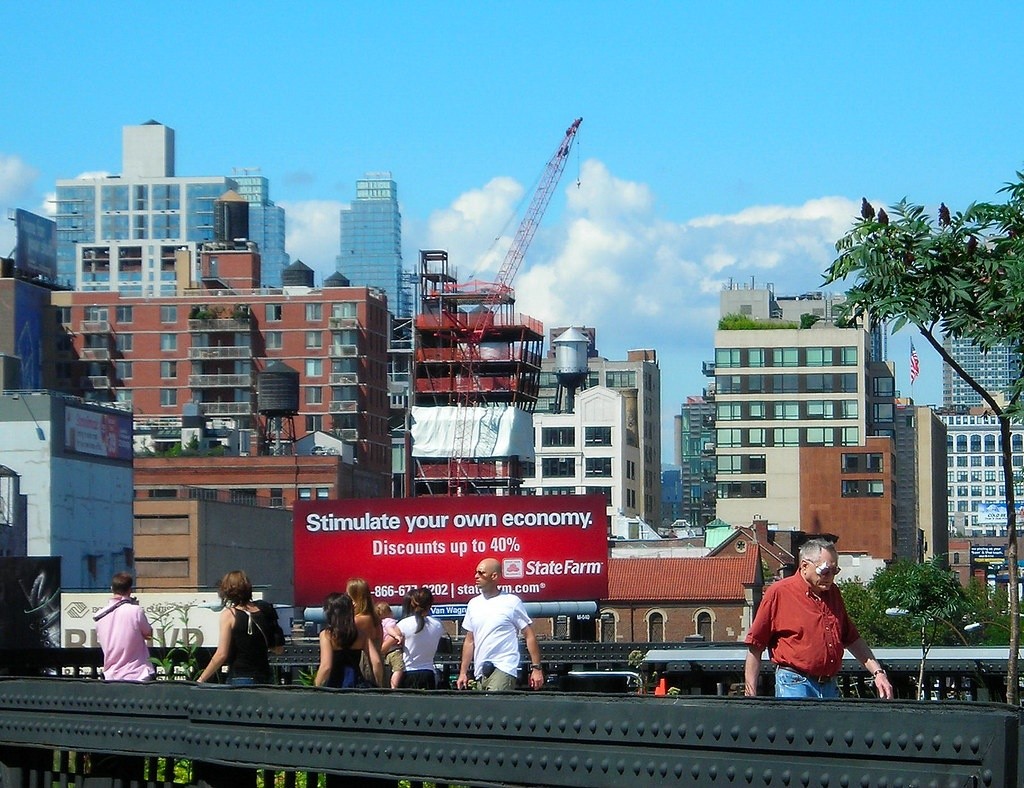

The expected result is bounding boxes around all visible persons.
[457,558,544,691]
[196,571,285,685]
[314,578,444,689]
[744,540,894,700]
[92,573,155,682]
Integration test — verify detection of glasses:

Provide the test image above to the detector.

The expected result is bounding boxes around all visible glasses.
[805,559,841,575]
[475,571,494,576]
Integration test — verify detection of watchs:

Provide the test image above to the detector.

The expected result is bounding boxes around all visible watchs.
[874,670,886,677]
[530,663,543,670]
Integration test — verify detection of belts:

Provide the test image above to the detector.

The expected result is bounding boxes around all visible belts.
[779,664,833,683]
[227,677,254,679]
